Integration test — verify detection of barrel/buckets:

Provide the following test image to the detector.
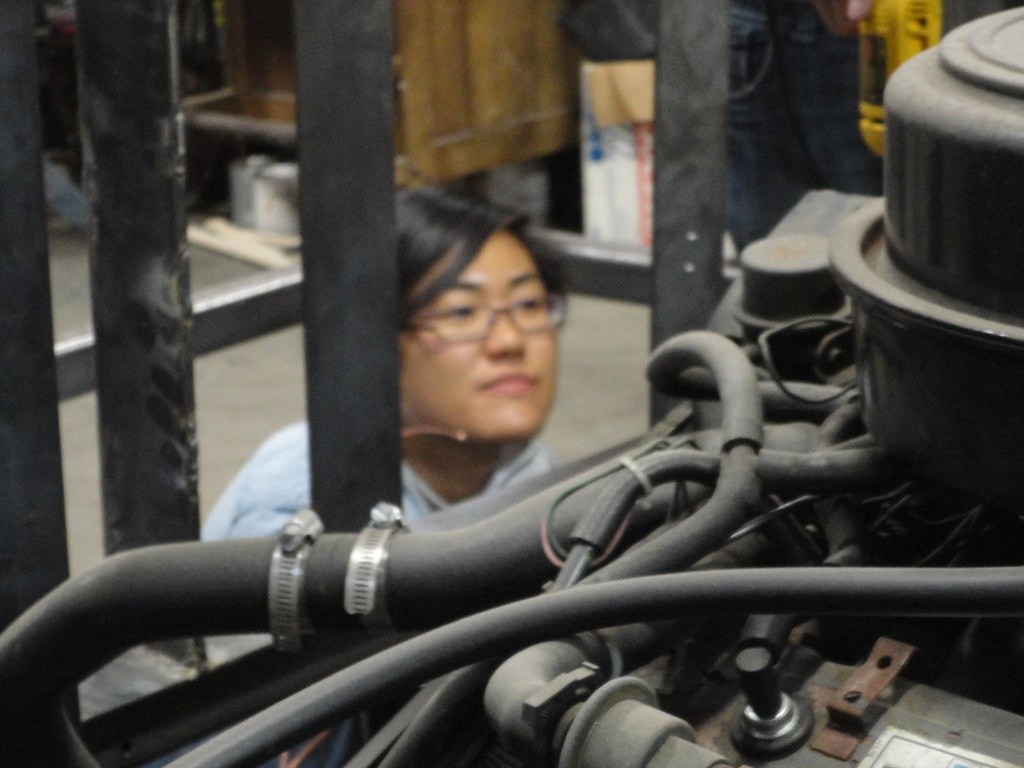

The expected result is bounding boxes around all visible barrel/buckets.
[250,160,300,240]
[230,155,270,225]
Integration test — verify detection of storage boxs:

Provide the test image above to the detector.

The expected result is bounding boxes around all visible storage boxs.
[577,58,660,247]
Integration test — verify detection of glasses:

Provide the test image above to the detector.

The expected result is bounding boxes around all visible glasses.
[397,293,565,343]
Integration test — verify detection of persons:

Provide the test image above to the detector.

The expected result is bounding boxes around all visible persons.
[726,0,880,252]
[205,187,558,664]
